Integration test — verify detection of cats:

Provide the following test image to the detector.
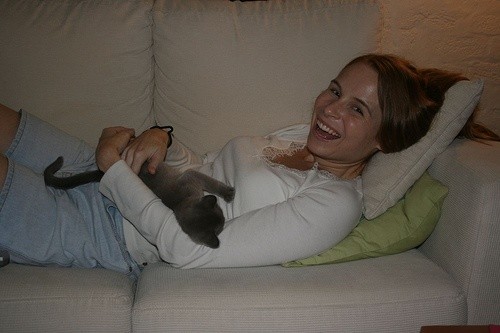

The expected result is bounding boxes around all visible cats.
[43,135,236,249]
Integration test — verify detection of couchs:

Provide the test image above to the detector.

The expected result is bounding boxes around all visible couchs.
[0,0,500,333]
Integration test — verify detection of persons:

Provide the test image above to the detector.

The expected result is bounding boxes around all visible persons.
[0,53,500,276]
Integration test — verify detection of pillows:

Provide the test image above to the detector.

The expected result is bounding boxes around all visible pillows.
[282,173,449,269]
[362,77,485,220]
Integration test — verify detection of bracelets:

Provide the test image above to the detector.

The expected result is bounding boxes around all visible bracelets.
[149,125,173,163]
